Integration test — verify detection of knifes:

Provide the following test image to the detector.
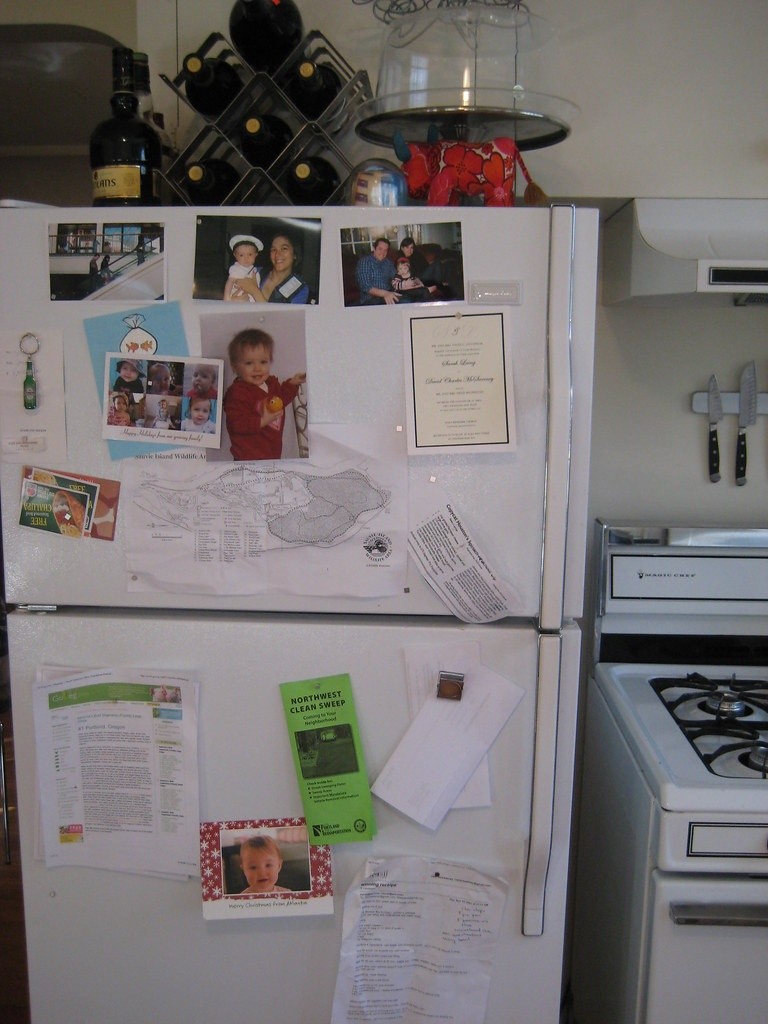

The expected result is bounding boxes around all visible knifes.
[707,361,755,486]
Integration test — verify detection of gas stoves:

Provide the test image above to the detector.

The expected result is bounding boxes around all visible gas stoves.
[595,519,767,877]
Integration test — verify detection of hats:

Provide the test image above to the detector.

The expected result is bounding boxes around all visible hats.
[116,358,146,378]
[229,235,264,252]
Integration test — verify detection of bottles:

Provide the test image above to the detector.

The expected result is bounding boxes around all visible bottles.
[285,61,349,140]
[237,115,298,180]
[88,45,176,207]
[185,160,260,207]
[183,57,253,123]
[228,1,306,71]
[286,153,345,204]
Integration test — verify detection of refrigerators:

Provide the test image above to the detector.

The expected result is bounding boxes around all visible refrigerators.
[0,206,598,1024]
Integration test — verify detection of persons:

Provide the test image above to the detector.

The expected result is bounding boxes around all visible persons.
[135,241,145,265]
[238,835,292,894]
[89,253,100,292]
[354,237,450,308]
[101,254,113,284]
[223,329,306,462]
[223,232,310,304]
[106,357,218,435]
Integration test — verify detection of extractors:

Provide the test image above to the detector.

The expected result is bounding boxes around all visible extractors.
[603,196,767,310]
